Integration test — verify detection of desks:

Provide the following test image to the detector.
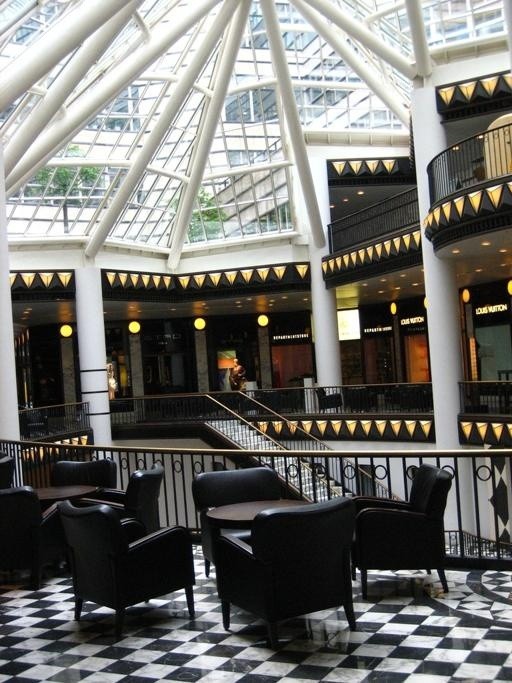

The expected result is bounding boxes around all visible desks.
[33,483,101,505]
[204,499,314,528]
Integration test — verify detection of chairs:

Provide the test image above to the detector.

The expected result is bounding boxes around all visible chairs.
[351,464,454,602]
[2,486,66,590]
[51,457,118,487]
[57,499,197,637]
[190,466,282,580]
[80,461,164,538]
[1,454,15,489]
[241,385,432,414]
[214,495,357,651]
[26,408,49,438]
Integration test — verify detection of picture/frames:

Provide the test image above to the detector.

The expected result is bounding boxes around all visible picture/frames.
[340,339,363,379]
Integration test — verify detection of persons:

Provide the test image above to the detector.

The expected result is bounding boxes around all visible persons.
[228,358,246,408]
